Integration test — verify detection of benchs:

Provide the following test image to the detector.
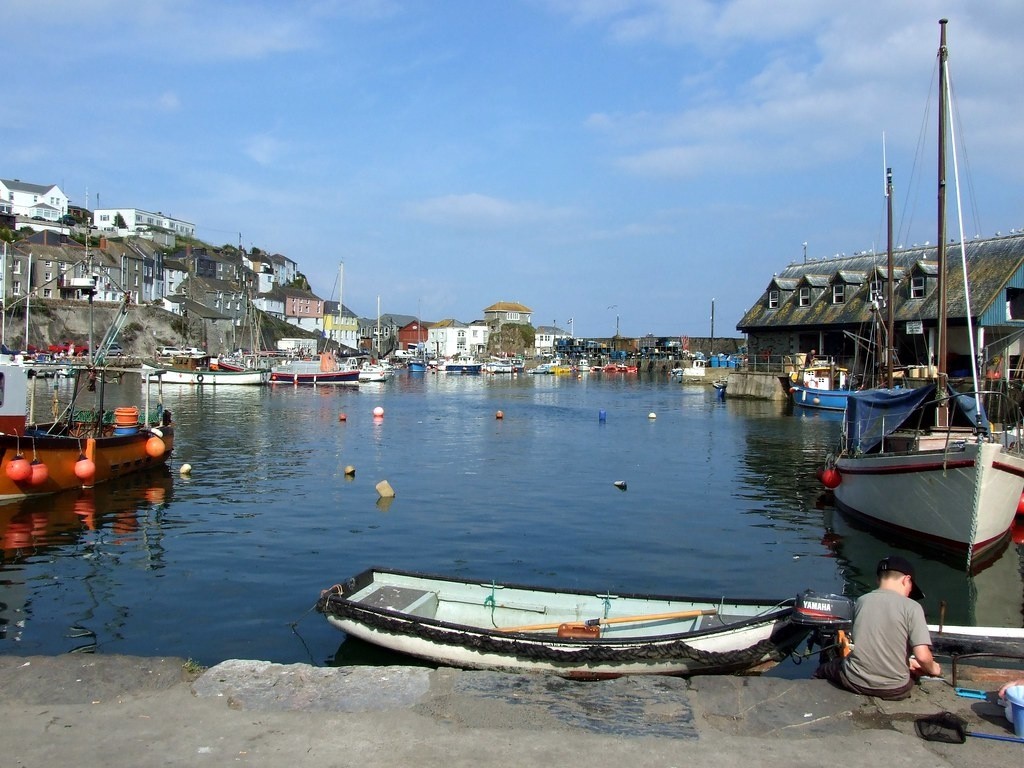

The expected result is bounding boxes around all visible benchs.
[66,408,157,438]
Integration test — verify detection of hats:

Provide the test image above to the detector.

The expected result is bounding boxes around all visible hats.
[877,556,926,601]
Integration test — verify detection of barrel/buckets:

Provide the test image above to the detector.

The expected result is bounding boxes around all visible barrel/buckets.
[719,356,727,367]
[711,355,719,367]
[1006,686,1024,737]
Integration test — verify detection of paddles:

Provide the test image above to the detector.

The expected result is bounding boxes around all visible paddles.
[485,607,721,636]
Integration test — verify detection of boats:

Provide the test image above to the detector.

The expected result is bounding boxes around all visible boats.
[811,611,1024,658]
[1,261,638,493]
[320,568,853,681]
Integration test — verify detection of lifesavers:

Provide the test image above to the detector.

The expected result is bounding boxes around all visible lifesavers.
[197,375,204,383]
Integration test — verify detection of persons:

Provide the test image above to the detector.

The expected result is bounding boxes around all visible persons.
[814,555,941,701]
[162,410,172,427]
[53,343,84,359]
[805,349,816,367]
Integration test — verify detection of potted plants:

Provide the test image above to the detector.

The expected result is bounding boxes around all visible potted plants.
[982,347,1002,378]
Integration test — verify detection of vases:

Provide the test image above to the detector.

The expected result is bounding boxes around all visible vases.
[909,366,920,377]
[930,366,937,378]
[920,366,928,377]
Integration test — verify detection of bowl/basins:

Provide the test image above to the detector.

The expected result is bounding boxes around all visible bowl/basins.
[114,406,139,435]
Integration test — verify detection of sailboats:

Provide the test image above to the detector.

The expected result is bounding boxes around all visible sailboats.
[780,16,1024,580]
